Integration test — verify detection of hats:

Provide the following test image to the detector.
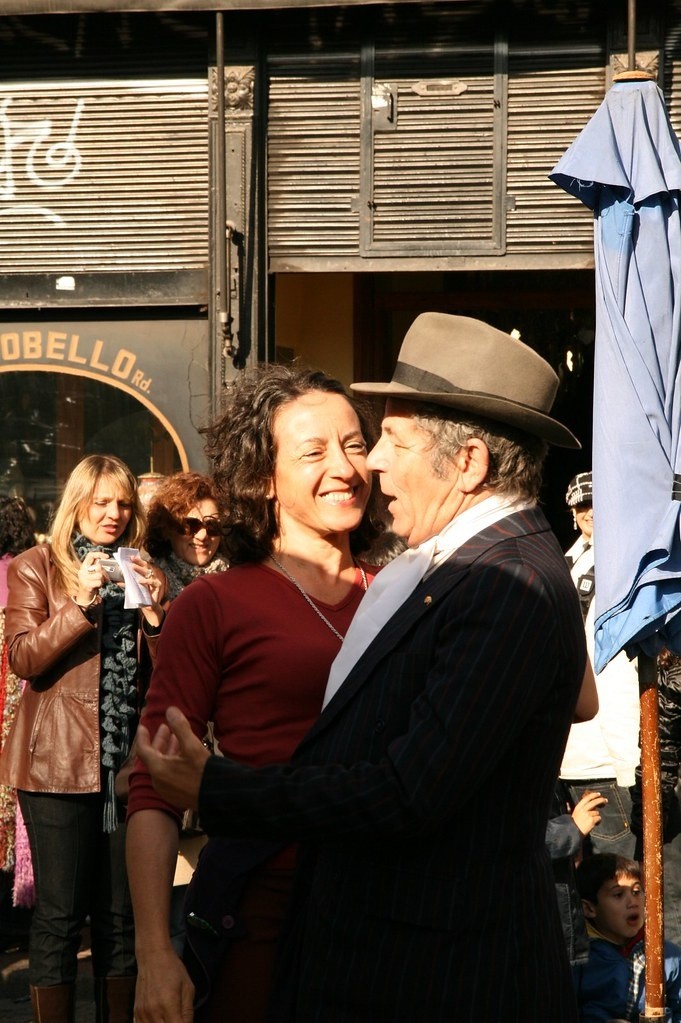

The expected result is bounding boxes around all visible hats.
[348,310,582,450]
[566,470,593,506]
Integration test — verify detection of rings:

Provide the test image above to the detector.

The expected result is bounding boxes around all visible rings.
[147,569,155,580]
[88,566,95,572]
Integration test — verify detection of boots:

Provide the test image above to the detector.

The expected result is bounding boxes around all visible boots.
[29,984,72,1023]
[94,976,137,1023]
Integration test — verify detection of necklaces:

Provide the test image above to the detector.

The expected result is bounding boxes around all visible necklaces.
[270,549,369,643]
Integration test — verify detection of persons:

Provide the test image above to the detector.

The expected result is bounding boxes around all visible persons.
[124,311,681,1023]
[0,455,167,1023]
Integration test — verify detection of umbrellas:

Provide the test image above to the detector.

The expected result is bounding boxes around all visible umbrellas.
[546,70,681,1022]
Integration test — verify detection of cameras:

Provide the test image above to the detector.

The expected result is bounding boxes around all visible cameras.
[90,559,124,583]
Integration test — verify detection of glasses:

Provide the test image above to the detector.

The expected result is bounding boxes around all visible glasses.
[173,516,228,537]
